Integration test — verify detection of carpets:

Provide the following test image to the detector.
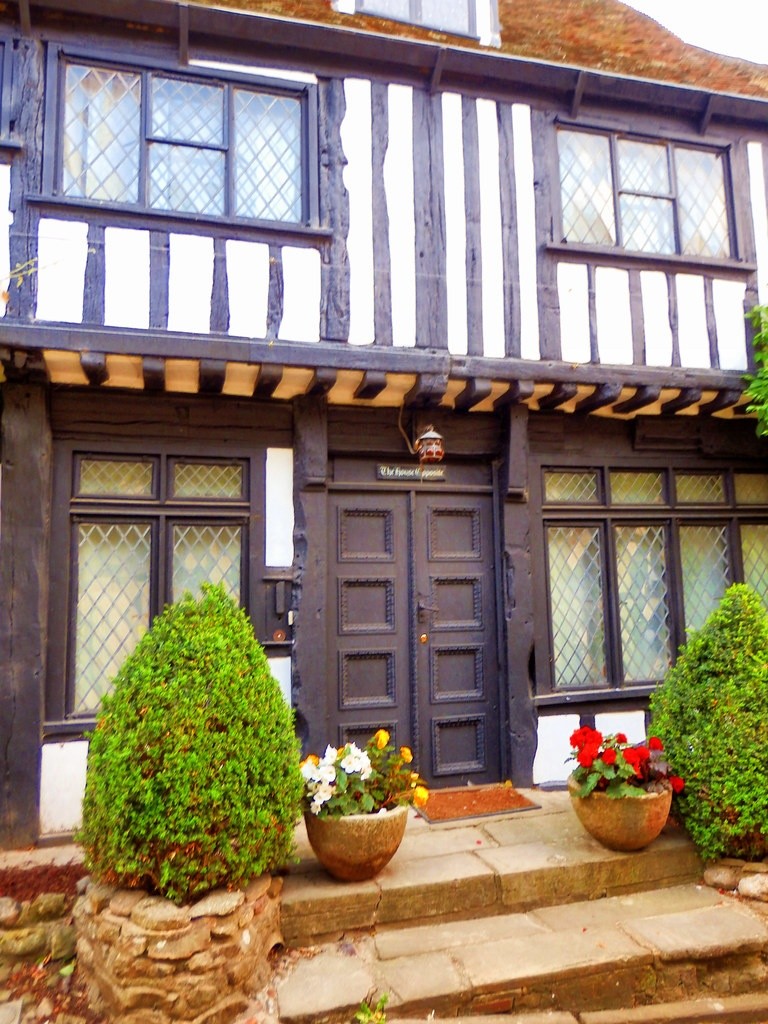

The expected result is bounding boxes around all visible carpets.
[411,784,544,824]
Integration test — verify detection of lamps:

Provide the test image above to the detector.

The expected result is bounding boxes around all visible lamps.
[417,423,444,461]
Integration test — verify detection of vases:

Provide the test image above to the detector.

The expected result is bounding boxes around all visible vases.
[567,770,674,852]
[303,803,408,883]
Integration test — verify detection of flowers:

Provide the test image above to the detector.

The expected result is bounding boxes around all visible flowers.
[565,726,688,800]
[297,727,430,822]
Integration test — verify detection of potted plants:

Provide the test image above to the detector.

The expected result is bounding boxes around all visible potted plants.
[73,581,306,1024]
